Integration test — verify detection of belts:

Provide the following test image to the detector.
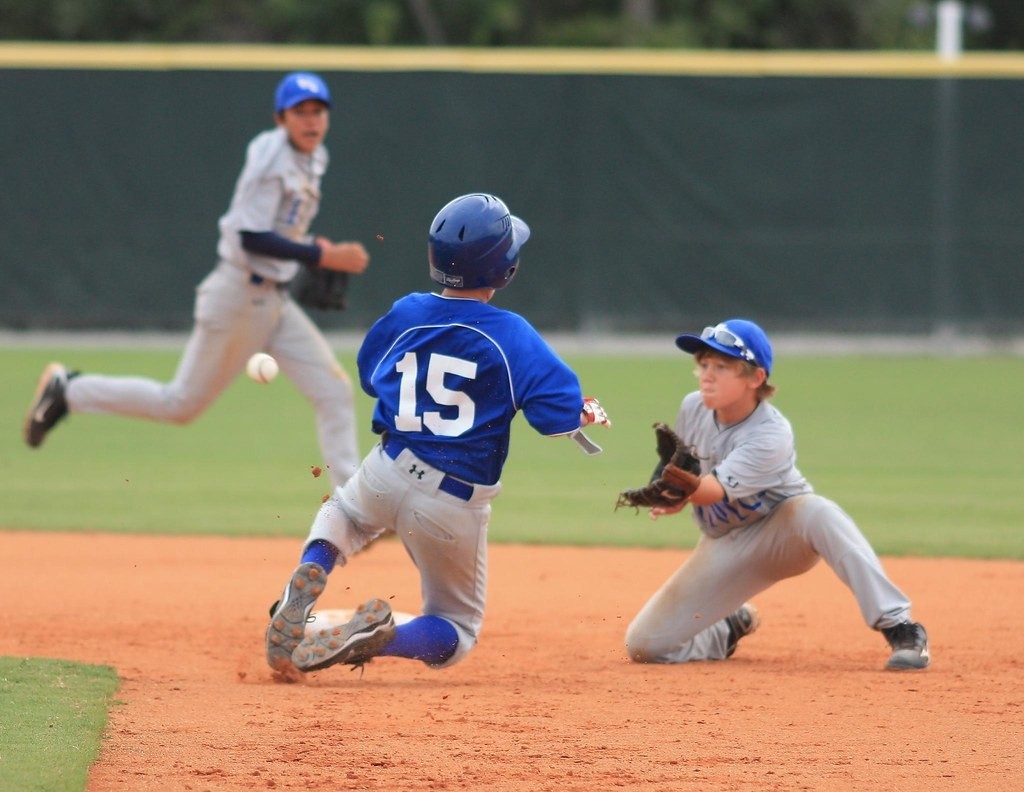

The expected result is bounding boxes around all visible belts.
[380,435,474,501]
[248,274,292,293]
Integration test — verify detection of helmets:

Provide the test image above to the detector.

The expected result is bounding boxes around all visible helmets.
[426,192,533,289]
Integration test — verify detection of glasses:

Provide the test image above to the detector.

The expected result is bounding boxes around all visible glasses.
[700,325,761,368]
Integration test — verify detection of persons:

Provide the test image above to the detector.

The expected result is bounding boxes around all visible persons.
[263,190,612,684]
[614,318,928,672]
[26,70,371,489]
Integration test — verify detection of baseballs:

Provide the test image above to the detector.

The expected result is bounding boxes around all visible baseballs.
[246,352,279,383]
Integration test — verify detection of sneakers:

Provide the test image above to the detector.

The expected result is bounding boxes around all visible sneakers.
[20,362,80,448]
[264,562,327,671]
[726,603,759,657]
[882,621,930,669]
[293,599,396,676]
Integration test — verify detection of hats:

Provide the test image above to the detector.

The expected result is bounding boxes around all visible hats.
[273,73,331,111]
[675,319,772,377]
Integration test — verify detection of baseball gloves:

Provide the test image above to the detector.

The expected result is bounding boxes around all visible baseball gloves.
[614,424,702,517]
